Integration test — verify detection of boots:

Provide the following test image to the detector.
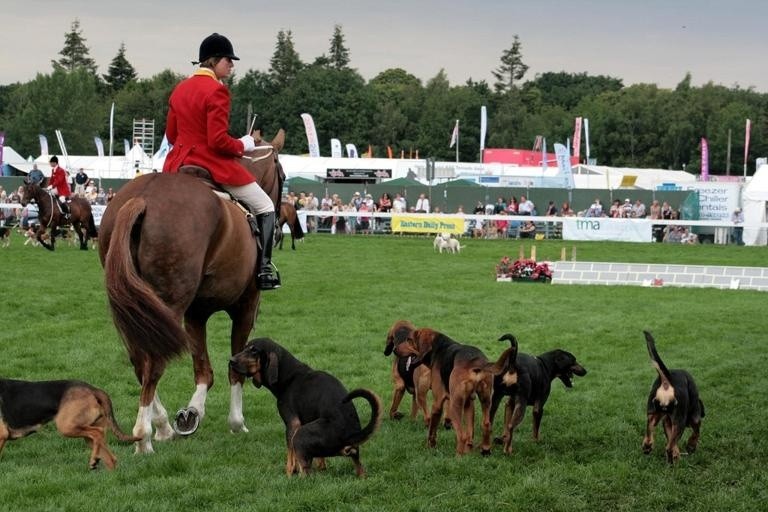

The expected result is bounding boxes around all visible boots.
[254,211,281,290]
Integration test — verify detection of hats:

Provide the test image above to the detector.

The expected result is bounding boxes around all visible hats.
[365,193,372,199]
[353,191,361,195]
[50,155,58,164]
[199,33,241,63]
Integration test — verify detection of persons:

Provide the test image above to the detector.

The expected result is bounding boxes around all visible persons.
[731,206,746,246]
[161,33,283,292]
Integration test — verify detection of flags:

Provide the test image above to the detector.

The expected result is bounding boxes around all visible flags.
[449,120,459,148]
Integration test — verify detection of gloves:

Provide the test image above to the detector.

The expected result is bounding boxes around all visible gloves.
[238,135,255,153]
[46,184,52,191]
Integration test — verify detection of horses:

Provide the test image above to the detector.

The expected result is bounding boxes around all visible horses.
[21,178,99,250]
[96,125,287,460]
[275,200,305,252]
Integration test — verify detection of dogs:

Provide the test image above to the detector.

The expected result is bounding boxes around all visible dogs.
[442,233,466,253]
[16,224,96,251]
[432,233,446,252]
[0,378,146,472]
[383,318,588,459]
[226,334,382,478]
[637,327,705,465]
[0,227,14,250]
